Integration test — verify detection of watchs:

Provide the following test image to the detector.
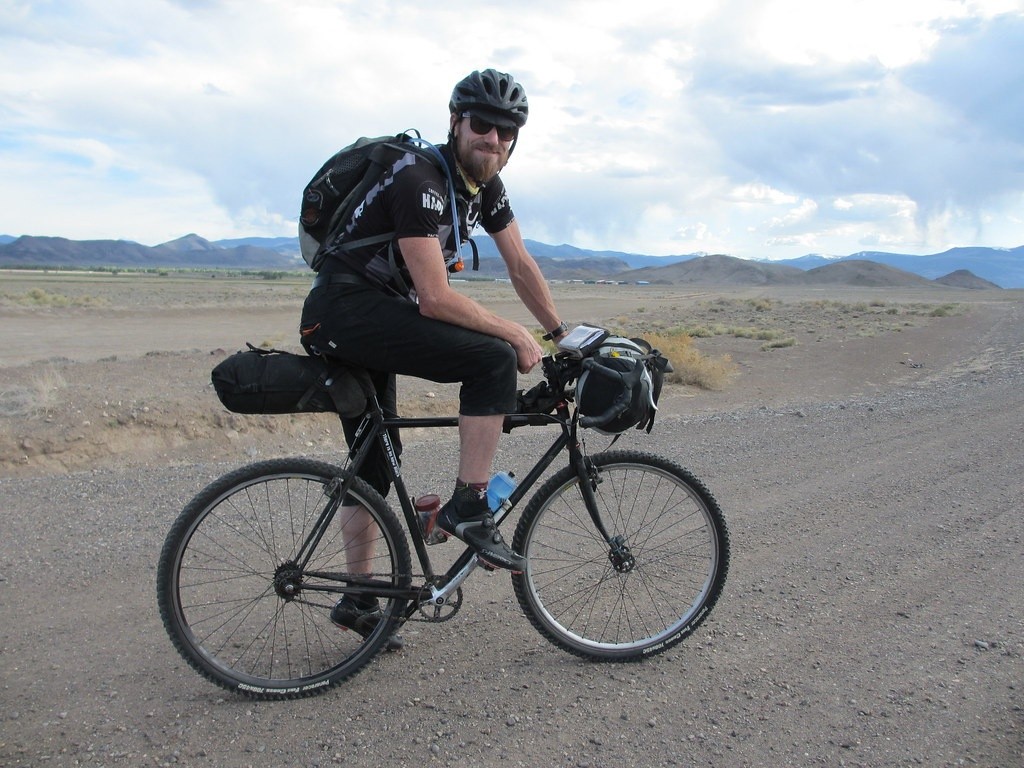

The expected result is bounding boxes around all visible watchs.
[542,321,569,342]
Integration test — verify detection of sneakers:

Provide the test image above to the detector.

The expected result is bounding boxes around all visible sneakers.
[434,499,528,574]
[330,593,404,651]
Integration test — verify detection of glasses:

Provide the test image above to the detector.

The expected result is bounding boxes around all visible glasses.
[461,111,518,141]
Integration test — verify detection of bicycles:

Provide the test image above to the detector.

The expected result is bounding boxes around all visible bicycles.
[156,345,732,704]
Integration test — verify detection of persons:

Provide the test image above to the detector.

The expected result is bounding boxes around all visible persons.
[299,69,570,651]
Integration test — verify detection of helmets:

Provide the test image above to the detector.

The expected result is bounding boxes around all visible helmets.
[449,69,528,129]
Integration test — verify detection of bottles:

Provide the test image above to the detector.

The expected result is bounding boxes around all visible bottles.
[487,471,516,512]
[415,494,447,545]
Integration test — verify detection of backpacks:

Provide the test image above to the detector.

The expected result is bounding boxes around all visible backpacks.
[299,129,487,294]
